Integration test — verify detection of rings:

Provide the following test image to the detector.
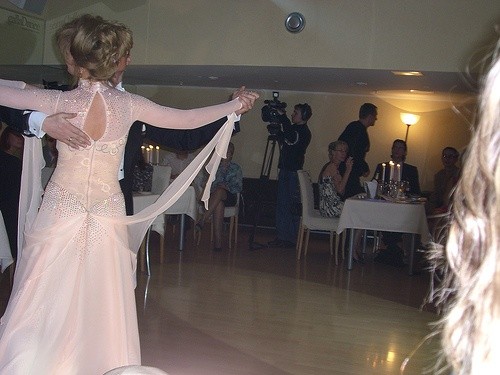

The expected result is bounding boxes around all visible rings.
[68,137,73,142]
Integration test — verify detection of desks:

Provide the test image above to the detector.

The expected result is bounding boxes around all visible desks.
[133,191,165,276]
[336,193,431,271]
[163,186,197,251]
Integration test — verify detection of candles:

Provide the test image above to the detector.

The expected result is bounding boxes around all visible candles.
[397,164,400,181]
[382,163,386,180]
[390,163,393,180]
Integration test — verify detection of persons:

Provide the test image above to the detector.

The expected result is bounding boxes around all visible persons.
[266,103,312,248]
[0,15,261,375]
[318,41,500,375]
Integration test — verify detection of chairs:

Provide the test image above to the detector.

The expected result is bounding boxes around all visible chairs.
[210,192,240,248]
[296,169,346,265]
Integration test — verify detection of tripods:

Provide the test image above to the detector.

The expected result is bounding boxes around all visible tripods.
[245,124,283,249]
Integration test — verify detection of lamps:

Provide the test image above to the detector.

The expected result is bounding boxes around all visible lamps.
[141,144,159,165]
[400,113,421,141]
